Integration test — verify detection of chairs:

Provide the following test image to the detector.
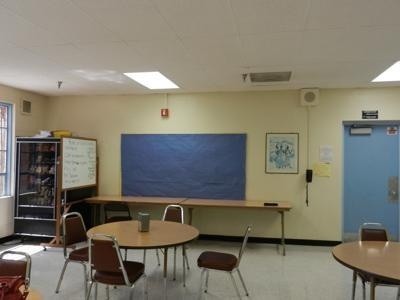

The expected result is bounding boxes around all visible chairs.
[197,226,251,300]
[0,249,31,300]
[86,234,145,300]
[103,203,132,223]
[357,222,400,300]
[55,212,97,300]
[155,205,190,271]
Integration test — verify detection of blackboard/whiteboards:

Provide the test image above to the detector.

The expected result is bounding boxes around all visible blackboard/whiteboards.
[60,135,97,192]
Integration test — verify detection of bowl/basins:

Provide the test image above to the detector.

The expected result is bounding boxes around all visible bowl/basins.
[52,130,73,138]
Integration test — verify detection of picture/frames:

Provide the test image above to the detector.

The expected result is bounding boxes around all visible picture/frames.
[265,132,299,174]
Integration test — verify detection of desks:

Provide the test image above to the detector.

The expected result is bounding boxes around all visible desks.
[85,196,188,227]
[86,220,200,300]
[179,198,293,256]
[332,241,400,300]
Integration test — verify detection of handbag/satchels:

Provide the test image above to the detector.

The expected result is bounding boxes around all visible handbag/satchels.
[0,272,30,300]
